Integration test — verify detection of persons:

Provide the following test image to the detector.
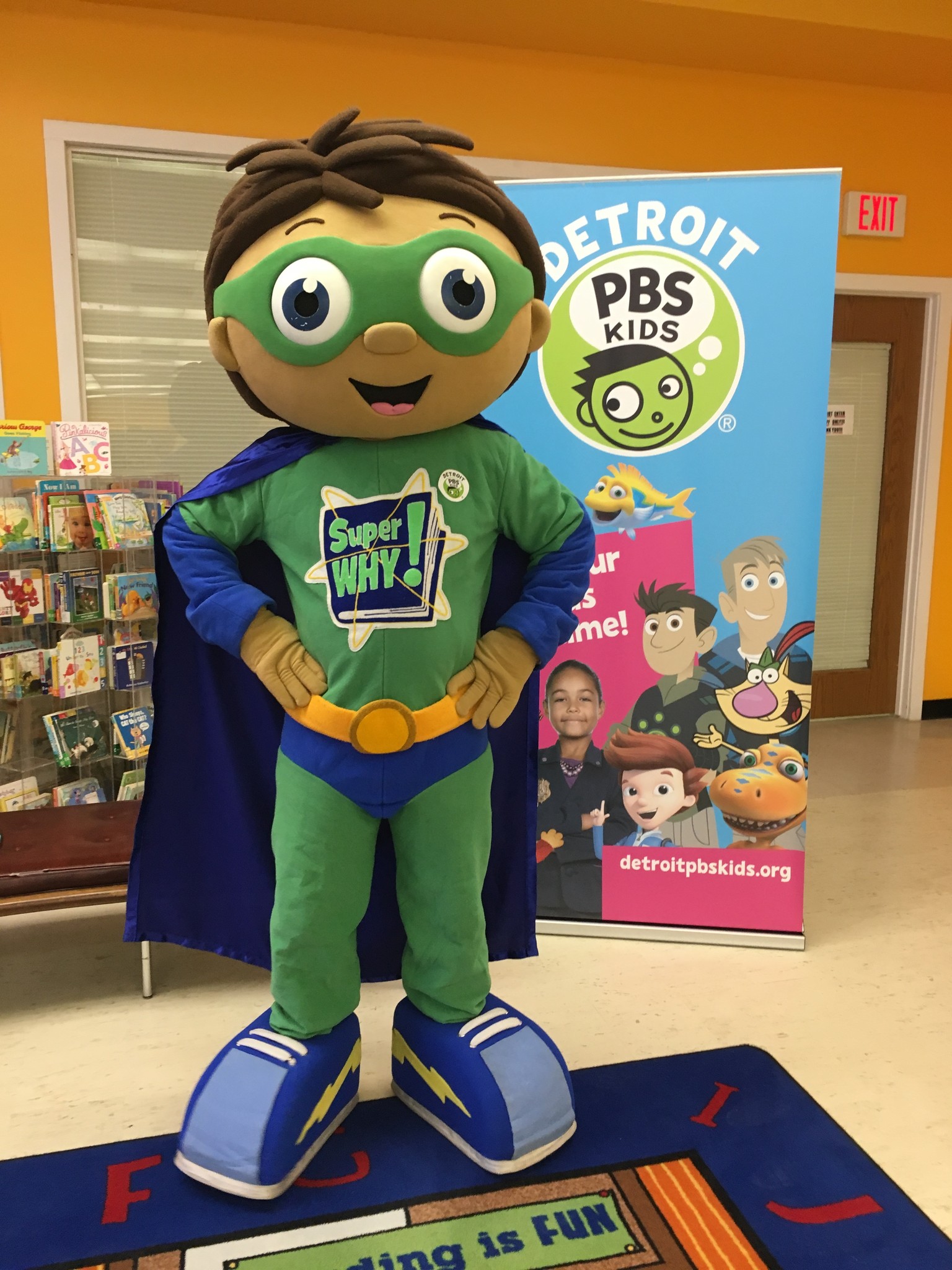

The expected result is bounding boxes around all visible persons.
[114,102,591,1206]
[518,657,628,920]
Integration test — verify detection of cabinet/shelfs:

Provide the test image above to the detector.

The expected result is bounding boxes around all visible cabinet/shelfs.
[0,471,192,998]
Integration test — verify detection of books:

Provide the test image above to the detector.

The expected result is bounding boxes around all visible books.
[0,417,193,816]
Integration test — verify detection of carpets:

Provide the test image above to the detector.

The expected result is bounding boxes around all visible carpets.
[3,1044,951,1268]
